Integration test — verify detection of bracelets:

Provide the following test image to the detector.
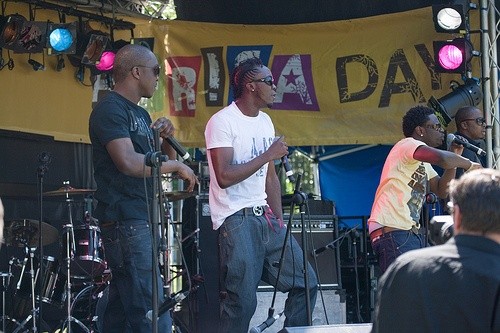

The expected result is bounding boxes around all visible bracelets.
[463,161,473,173]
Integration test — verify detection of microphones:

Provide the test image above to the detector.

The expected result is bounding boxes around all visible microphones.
[145,291,189,323]
[250,312,282,333]
[446,133,487,157]
[427,193,440,204]
[292,194,307,206]
[350,229,360,239]
[311,245,329,257]
[155,123,192,163]
[273,136,295,183]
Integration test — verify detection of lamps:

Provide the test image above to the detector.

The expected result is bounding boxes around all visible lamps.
[0,8,155,91]
[427,1,483,127]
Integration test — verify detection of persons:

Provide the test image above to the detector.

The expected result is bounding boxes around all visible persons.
[88,44,199,333]
[371,168,500,333]
[203,57,317,333]
[367,105,481,272]
[448,106,488,179]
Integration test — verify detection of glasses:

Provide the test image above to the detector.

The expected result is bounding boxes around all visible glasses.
[422,123,441,131]
[250,76,275,87]
[461,117,486,126]
[130,65,161,77]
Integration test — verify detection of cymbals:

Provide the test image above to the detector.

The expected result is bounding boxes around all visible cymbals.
[2,218,60,249]
[155,189,199,202]
[41,185,96,198]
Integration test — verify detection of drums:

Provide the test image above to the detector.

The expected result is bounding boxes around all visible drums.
[58,224,107,280]
[65,282,110,332]
[14,254,67,310]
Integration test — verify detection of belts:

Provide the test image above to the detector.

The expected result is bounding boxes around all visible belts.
[370,227,401,241]
[235,205,275,217]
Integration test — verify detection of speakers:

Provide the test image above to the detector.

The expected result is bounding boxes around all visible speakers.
[182,196,220,333]
[256,232,339,291]
